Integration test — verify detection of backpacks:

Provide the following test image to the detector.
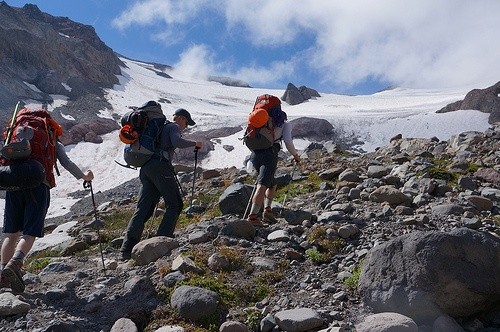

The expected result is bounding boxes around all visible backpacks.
[0,107,62,191]
[114,100,167,170]
[239,94,282,151]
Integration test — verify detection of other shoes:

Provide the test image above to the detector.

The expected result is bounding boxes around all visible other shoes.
[2,260,25,295]
[0,269,13,288]
[118,250,131,260]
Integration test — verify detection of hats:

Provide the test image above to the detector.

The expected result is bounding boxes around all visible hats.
[173,108,196,126]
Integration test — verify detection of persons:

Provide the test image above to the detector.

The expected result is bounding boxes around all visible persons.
[118,108,202,260]
[0,109,94,292]
[247,99,301,227]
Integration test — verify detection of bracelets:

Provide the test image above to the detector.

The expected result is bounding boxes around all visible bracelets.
[293,154,298,156]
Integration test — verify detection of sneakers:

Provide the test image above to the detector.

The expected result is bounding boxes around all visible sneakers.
[248,214,264,228]
[262,207,279,223]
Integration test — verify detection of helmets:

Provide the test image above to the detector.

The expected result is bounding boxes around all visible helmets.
[119,124,138,144]
[249,109,269,128]
[0,137,32,162]
